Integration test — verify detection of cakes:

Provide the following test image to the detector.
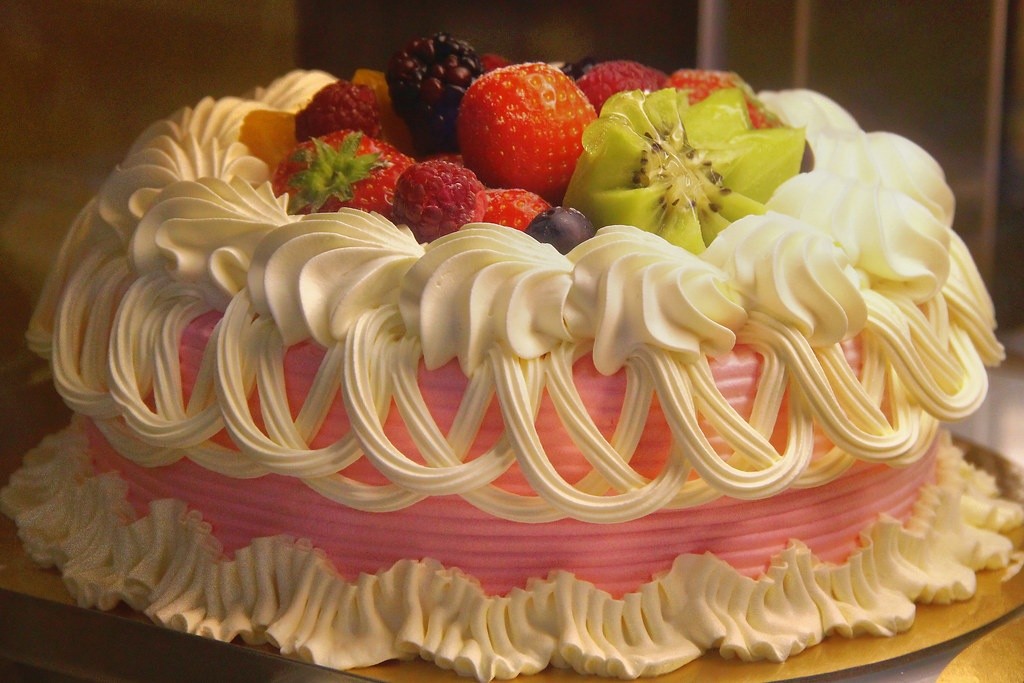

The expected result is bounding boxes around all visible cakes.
[2,33,1023,679]
